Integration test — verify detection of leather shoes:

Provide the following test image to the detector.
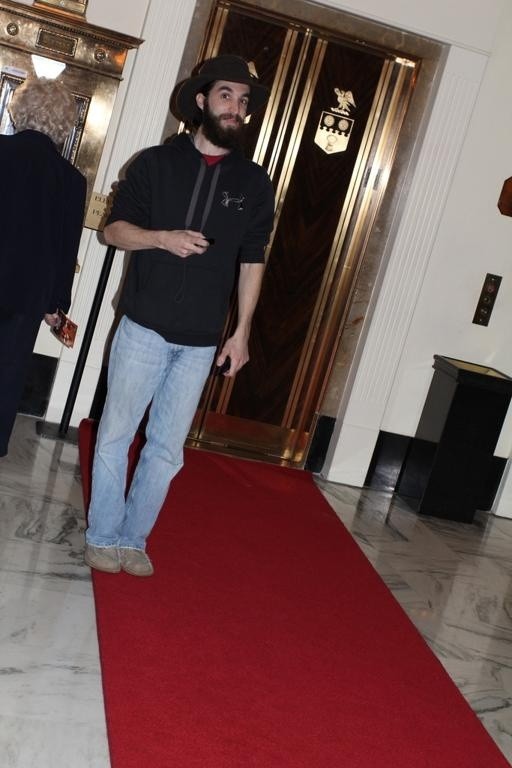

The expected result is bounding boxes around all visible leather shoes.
[121,548,155,578]
[85,543,121,573]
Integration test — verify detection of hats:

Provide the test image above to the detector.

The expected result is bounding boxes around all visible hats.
[170,55,271,126]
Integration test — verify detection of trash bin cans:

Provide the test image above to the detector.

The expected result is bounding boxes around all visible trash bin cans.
[395,354,512,524]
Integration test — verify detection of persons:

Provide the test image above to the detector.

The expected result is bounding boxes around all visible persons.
[82,54,277,578]
[0,74,89,460]
